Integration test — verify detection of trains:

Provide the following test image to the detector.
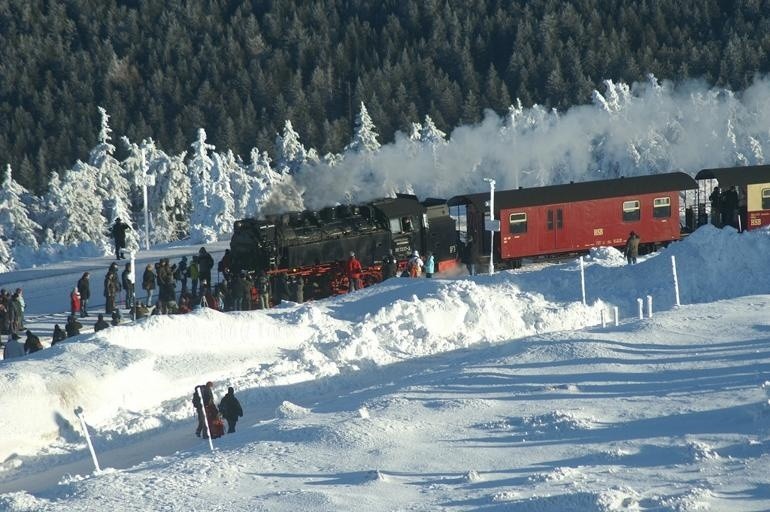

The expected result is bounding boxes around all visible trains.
[227,164,770,301]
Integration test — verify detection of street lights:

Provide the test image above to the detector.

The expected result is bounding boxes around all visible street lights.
[481,177,496,276]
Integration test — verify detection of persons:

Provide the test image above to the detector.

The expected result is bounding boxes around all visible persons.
[105,247,306,326]
[408,250,424,278]
[344,255,365,294]
[195,382,219,439]
[3,333,25,359]
[461,233,480,276]
[219,386,246,434]
[71,272,90,317]
[109,217,129,260]
[24,330,43,354]
[423,250,435,278]
[95,313,109,332]
[401,262,418,278]
[383,255,398,282]
[0,287,27,338]
[623,230,641,266]
[51,325,66,346]
[707,185,747,233]
[66,316,82,338]
